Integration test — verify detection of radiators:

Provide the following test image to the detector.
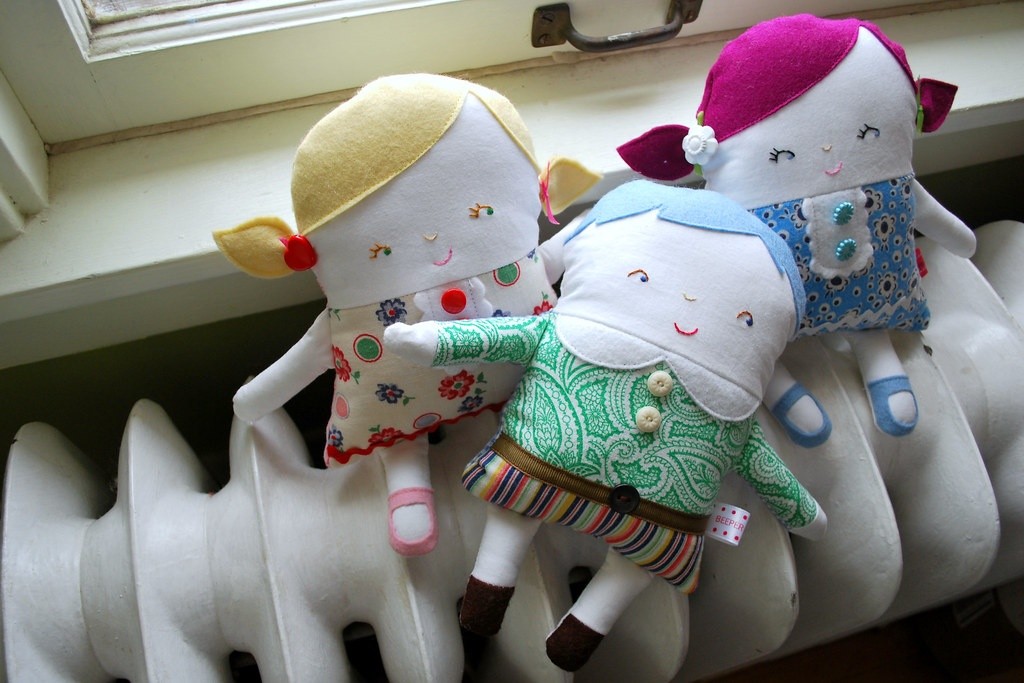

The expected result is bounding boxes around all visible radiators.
[0,219,1024,683]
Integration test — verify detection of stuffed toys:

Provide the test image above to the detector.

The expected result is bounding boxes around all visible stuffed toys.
[384,179,829,673]
[617,14,977,449]
[211,73,604,558]
